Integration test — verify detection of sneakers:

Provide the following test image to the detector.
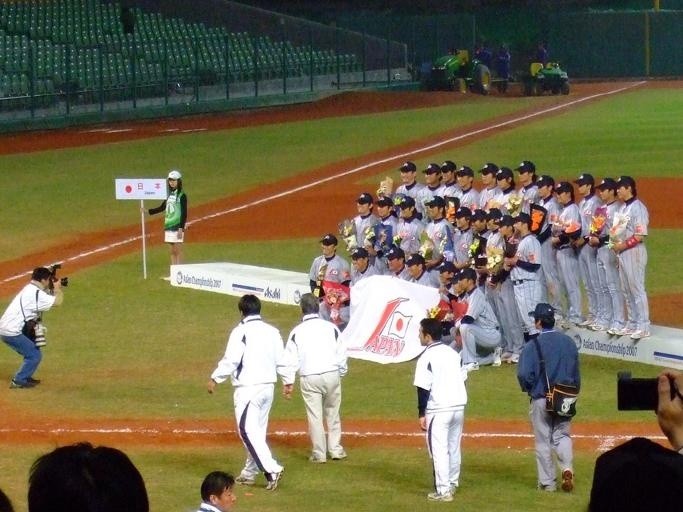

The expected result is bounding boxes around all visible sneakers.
[630,330,652,340]
[307,452,327,463]
[554,313,607,331]
[560,467,575,493]
[234,473,257,487]
[425,480,460,503]
[607,326,634,336]
[490,346,503,367]
[500,351,520,364]
[461,361,479,372]
[163,274,171,284]
[534,481,559,493]
[329,446,348,461]
[263,465,285,491]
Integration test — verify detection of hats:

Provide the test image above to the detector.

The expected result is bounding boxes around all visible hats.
[448,206,532,228]
[397,196,415,208]
[397,161,417,173]
[514,160,536,174]
[383,246,405,262]
[318,232,337,246]
[421,159,474,179]
[354,192,373,206]
[478,163,514,181]
[347,246,368,258]
[527,302,557,320]
[423,195,446,208]
[374,196,393,207]
[166,170,183,182]
[436,261,479,285]
[404,253,426,267]
[534,172,635,196]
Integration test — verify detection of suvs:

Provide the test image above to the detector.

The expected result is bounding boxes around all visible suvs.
[422,49,510,93]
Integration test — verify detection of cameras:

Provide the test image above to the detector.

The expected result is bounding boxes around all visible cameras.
[49,264,68,289]
[618,377,676,411]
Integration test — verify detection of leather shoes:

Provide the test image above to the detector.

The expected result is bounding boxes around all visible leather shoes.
[8,376,41,389]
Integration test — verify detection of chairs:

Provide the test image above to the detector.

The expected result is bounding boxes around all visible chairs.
[1,0,362,99]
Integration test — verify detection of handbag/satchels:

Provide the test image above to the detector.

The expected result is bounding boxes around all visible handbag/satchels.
[543,382,579,420]
[22,319,37,342]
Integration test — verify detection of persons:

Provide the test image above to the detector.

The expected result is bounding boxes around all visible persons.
[585,369,682,512]
[474,41,547,95]
[282,292,348,463]
[412,317,468,502]
[517,304,580,491]
[206,294,296,492]
[139,170,187,281]
[194,471,237,512]
[0,267,64,389]
[27,442,149,512]
